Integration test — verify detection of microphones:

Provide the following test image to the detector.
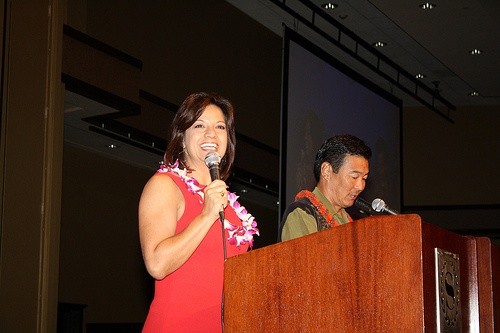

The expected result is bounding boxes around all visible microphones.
[204,152,225,221]
[372,198,400,215]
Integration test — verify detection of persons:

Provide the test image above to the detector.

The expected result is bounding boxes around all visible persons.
[138,92,259,333]
[277,134,373,242]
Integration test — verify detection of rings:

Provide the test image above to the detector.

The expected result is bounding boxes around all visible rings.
[220,192,224,197]
